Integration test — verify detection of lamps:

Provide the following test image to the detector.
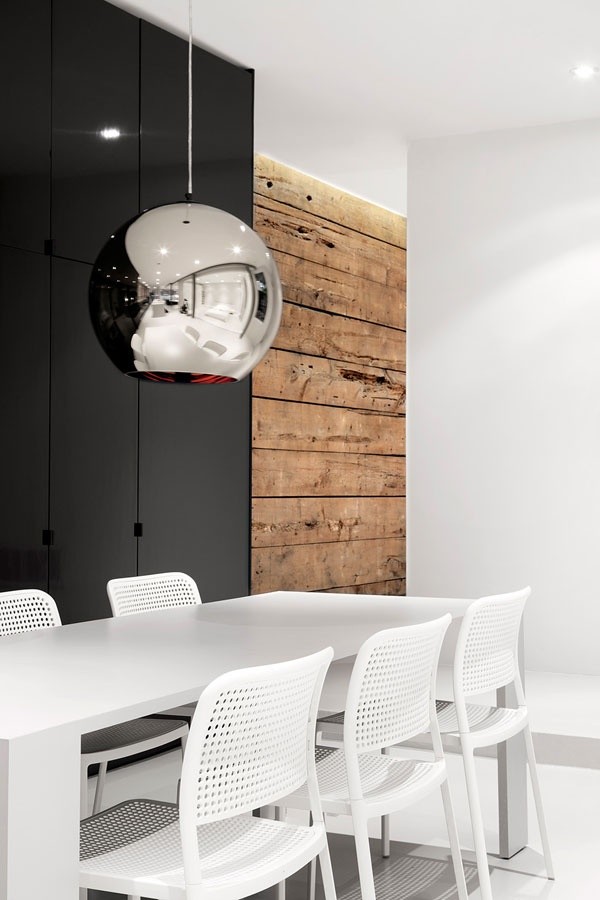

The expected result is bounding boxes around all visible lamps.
[84,1,283,386]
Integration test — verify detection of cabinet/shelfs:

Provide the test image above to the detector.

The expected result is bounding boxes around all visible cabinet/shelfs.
[0,2,253,627]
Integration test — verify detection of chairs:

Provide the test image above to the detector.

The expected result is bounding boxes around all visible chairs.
[317,583,556,900]
[78,646,340,900]
[129,324,252,381]
[0,589,187,816]
[106,570,205,717]
[175,614,473,900]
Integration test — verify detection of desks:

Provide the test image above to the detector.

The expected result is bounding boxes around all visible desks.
[0,591,528,900]
[150,297,167,317]
